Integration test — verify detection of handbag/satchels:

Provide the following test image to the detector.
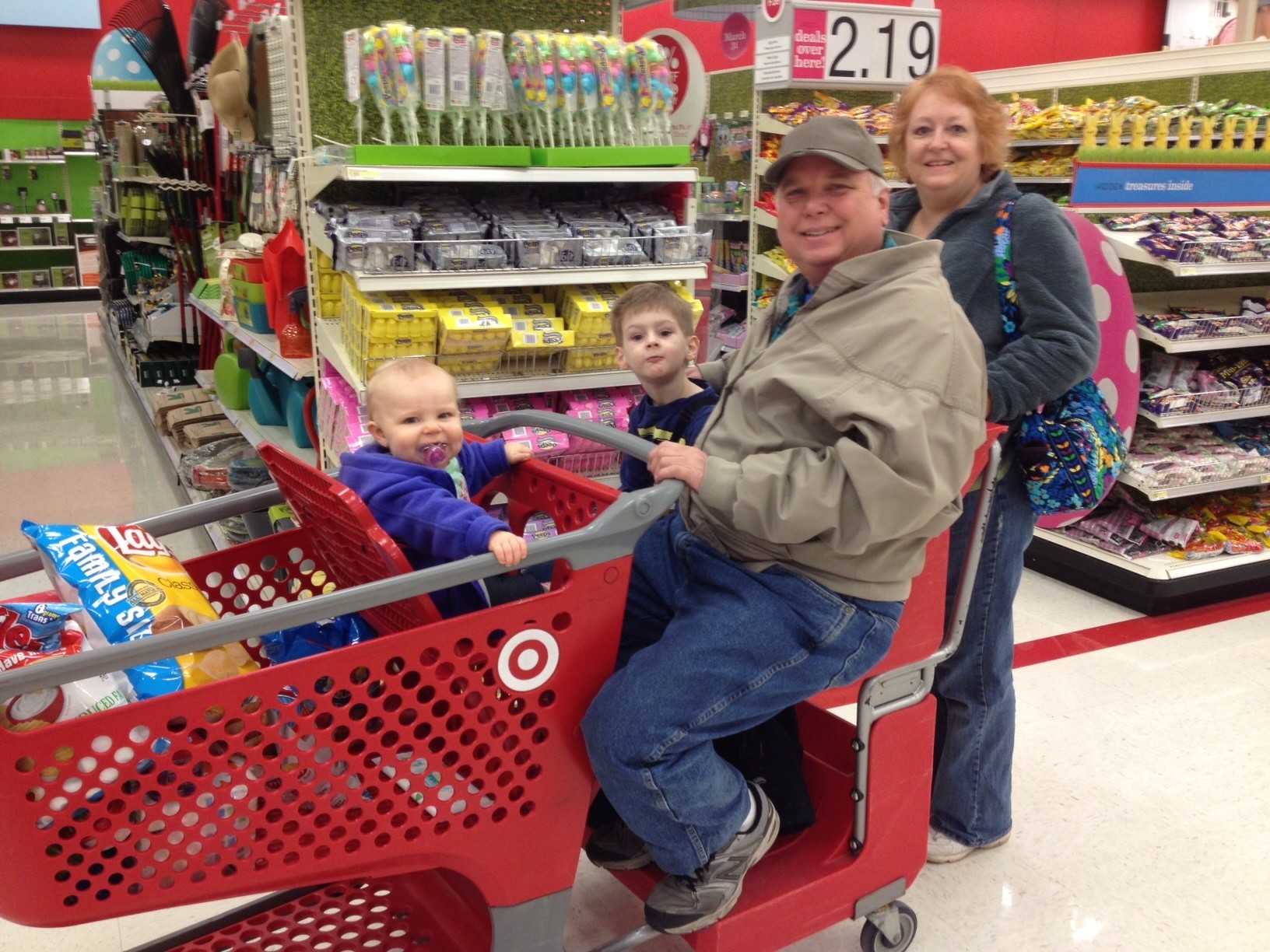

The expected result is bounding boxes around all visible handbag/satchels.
[996,192,1128,517]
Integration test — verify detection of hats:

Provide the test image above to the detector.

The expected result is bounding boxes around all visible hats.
[765,114,886,183]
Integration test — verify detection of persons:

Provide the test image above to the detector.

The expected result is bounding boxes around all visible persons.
[881,65,1130,863]
[338,357,540,621]
[578,115,986,934]
[611,283,721,494]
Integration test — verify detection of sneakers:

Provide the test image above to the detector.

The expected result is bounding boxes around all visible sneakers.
[586,820,652,871]
[643,787,779,935]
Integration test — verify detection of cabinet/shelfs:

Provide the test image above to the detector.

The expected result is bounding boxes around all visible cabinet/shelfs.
[0,136,310,555]
[282,0,711,478]
[700,36,1270,617]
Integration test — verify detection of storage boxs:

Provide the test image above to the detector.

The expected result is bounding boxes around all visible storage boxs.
[314,245,704,391]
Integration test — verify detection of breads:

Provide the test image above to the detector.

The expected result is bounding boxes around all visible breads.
[149,605,250,681]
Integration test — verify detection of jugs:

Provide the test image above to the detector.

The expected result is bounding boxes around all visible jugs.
[236,347,318,448]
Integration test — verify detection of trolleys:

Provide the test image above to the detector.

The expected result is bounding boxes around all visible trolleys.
[0,399,1007,952]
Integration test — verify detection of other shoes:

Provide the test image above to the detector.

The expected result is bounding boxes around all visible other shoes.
[926,821,1010,863]
